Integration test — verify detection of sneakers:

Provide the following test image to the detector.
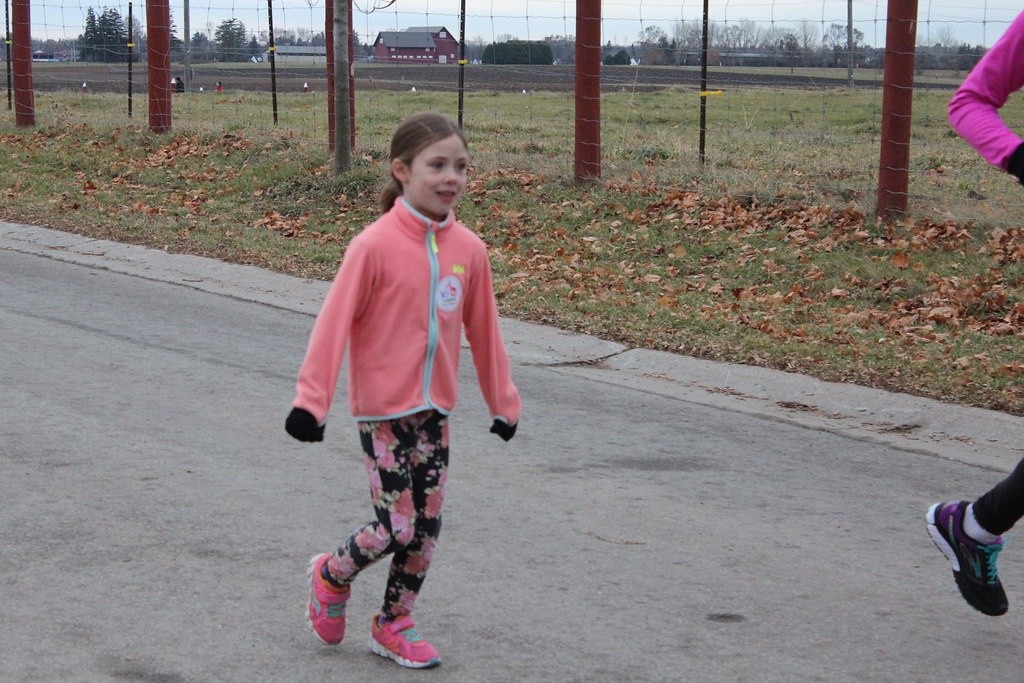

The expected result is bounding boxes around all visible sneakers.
[305,553,351,645]
[367,613,442,670]
[925,500,1009,616]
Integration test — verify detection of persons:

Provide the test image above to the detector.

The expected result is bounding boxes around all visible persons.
[283,111,522,669]
[216,81,223,91]
[176,76,184,93]
[925,9,1024,616]
